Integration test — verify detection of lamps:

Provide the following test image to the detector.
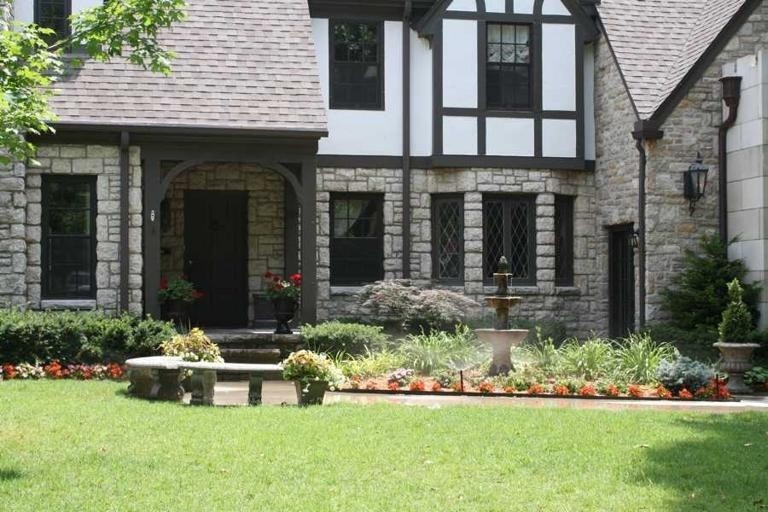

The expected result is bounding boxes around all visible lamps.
[684,152,709,216]
[627,229,640,248]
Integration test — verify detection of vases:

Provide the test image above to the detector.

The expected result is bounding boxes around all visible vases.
[295,379,330,407]
[182,370,196,393]
[164,299,188,327]
[271,296,298,333]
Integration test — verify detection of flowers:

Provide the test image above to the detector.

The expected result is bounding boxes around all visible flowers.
[153,328,224,363]
[278,347,349,391]
[260,271,302,298]
[158,272,201,302]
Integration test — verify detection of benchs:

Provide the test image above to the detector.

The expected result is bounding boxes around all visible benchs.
[125,356,185,399]
[179,356,287,406]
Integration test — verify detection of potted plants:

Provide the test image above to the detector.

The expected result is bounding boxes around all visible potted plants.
[713,277,761,393]
